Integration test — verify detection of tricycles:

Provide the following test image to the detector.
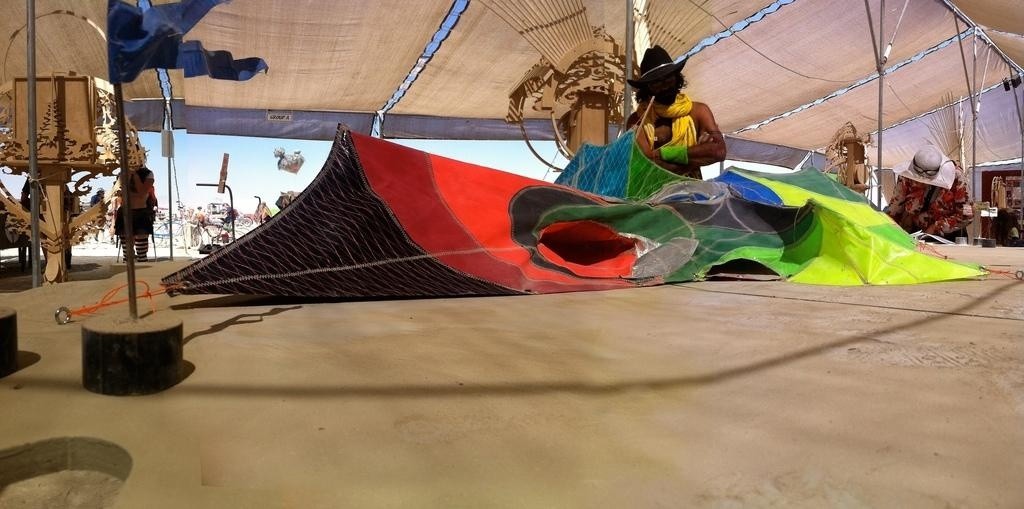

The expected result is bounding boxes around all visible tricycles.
[153,220,191,249]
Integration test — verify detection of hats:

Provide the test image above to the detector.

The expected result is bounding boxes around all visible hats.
[627,45,689,86]
[894,146,958,191]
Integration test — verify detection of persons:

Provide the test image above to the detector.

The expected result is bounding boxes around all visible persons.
[108,144,159,265]
[20,173,74,271]
[626,44,727,180]
[883,144,976,245]
[188,203,235,232]
[991,211,1019,246]
[90,187,107,242]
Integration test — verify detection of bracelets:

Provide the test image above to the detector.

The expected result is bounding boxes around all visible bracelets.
[659,145,689,168]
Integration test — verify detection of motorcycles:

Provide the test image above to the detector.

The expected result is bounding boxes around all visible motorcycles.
[236,215,253,227]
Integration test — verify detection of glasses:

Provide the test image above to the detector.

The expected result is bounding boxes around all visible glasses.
[913,157,941,176]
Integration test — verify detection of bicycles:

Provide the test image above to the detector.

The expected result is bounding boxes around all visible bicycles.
[189,219,234,249]
